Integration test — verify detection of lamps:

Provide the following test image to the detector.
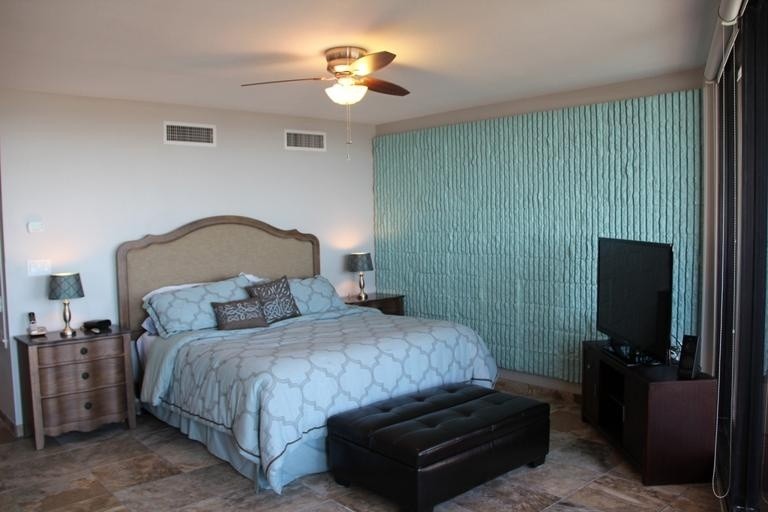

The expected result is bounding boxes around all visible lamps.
[48,272,85,336]
[346,250,374,300]
[318,46,369,108]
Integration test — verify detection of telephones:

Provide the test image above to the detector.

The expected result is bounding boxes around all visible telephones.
[26,324,47,337]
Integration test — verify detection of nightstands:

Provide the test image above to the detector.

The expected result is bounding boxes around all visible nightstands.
[14,325,135,449]
[341,291,405,315]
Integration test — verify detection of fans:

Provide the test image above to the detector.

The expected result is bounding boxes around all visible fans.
[239,46,411,97]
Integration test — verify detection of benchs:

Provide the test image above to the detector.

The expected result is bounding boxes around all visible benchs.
[325,381,551,510]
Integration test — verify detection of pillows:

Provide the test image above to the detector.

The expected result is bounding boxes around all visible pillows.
[138,271,353,340]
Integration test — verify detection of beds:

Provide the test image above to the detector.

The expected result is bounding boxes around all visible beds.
[114,214,497,495]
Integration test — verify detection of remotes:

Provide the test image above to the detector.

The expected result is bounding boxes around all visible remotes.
[91,327,101,334]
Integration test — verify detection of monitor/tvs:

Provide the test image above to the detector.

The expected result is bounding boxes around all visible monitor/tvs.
[596,236,673,369]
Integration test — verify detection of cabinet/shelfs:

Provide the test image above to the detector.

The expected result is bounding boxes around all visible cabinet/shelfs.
[580,338,719,487]
